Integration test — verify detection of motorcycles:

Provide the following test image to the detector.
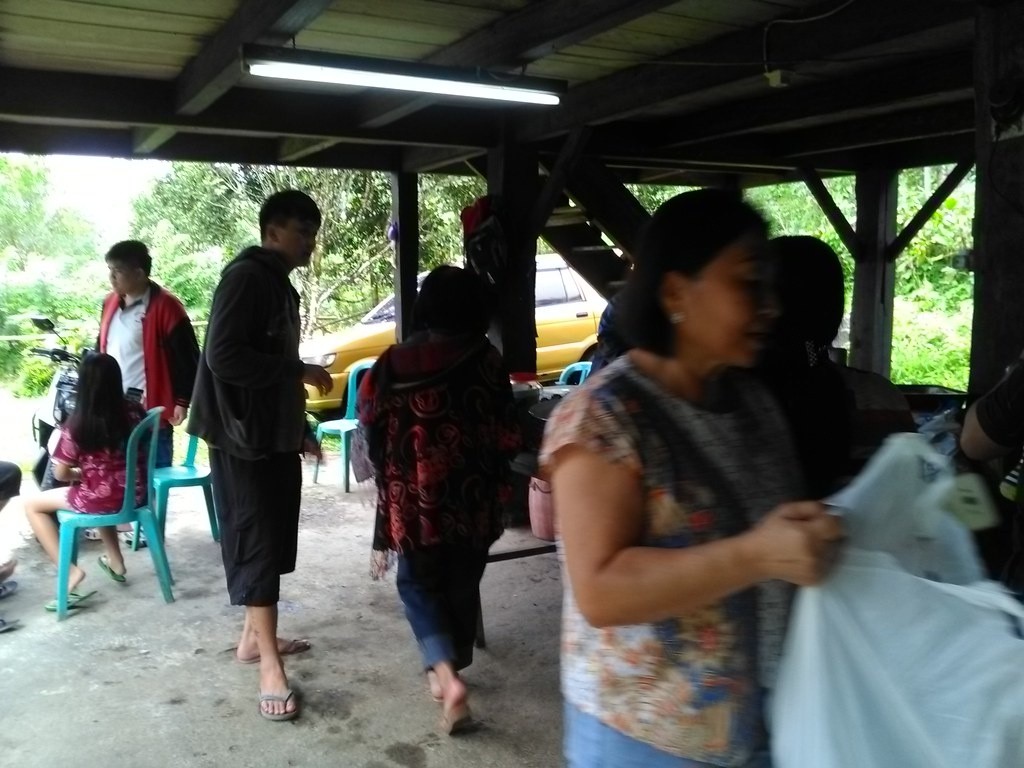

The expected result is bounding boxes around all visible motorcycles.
[27,315,96,492]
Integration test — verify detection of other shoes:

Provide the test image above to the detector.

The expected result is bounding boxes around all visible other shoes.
[86,529,101,541]
[120,527,147,547]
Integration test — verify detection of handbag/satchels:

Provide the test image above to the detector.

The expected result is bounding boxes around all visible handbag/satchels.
[770,431,1023,768]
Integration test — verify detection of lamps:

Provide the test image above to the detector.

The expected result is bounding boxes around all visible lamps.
[236,43,568,108]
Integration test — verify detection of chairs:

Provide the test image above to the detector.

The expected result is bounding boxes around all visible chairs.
[51,407,174,622]
[129,406,222,550]
[558,362,593,386]
[313,361,375,493]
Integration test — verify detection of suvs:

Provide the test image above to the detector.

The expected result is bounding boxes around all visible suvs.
[297,246,615,424]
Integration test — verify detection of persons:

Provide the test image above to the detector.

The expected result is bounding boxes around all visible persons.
[185,189,334,720]
[598,287,633,364]
[355,264,524,734]
[538,188,847,768]
[962,351,1024,575]
[0,461,23,634]
[25,352,148,611]
[94,239,201,547]
[765,236,854,502]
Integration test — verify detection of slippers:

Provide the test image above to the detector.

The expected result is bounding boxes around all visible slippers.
[239,638,310,663]
[258,687,298,721]
[443,712,472,737]
[0,618,20,632]
[0,580,17,598]
[45,591,96,611]
[98,555,127,582]
[431,685,445,703]
